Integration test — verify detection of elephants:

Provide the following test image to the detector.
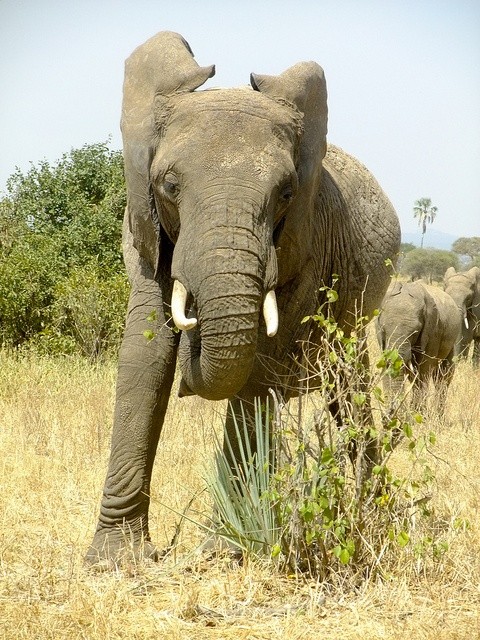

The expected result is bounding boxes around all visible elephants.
[374,278,457,418]
[82,31,401,574]
[442,267,480,370]
